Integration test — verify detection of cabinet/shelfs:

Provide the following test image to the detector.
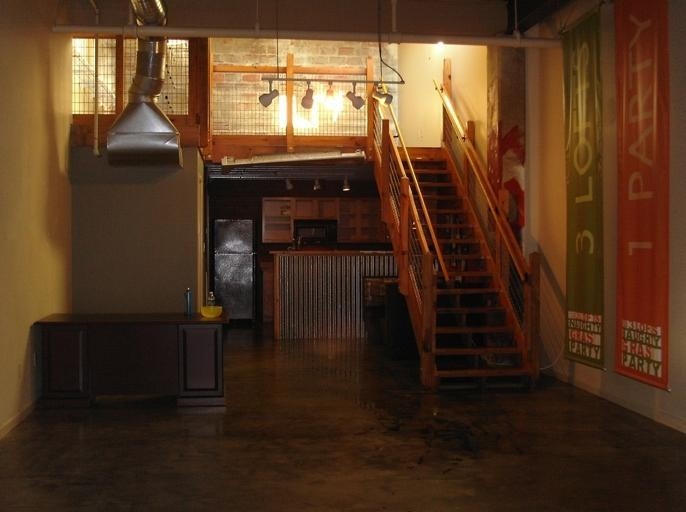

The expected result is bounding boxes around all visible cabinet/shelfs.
[208,291,216,307]
[183,287,192,317]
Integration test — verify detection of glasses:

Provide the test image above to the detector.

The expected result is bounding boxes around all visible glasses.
[259,0,405,110]
[283,178,350,193]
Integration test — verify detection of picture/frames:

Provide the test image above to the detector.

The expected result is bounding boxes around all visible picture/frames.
[32,311,229,415]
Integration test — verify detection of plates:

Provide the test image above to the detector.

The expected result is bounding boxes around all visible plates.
[262,197,295,244]
[295,197,339,220]
[326,198,384,244]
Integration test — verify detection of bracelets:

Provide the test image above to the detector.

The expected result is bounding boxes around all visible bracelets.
[199,306,223,318]
